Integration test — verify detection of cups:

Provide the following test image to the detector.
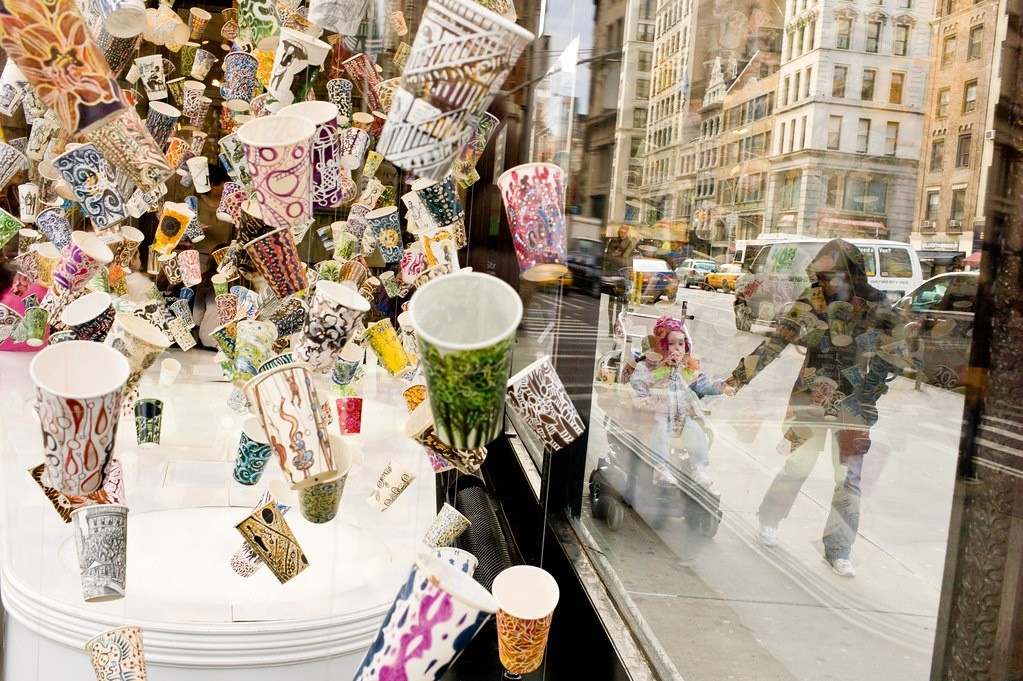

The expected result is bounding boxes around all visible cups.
[0,0,588,681]
[598,1,954,498]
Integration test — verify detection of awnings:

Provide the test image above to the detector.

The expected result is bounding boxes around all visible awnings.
[915,252,966,269]
[960,252,982,268]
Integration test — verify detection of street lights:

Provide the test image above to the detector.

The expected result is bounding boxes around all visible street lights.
[706,171,749,264]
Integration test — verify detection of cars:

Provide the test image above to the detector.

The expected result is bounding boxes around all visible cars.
[632,257,678,302]
[922,280,948,301]
[704,264,744,293]
[888,273,978,383]
[539,236,626,299]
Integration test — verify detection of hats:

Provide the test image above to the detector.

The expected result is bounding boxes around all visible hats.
[653,317,692,354]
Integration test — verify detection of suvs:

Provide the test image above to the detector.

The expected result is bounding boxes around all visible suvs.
[674,259,715,288]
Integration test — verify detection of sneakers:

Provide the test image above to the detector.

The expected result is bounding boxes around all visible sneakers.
[691,469,712,488]
[756,511,778,547]
[652,466,679,489]
[821,555,857,577]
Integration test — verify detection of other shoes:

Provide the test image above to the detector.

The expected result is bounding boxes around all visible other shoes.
[608,324,623,337]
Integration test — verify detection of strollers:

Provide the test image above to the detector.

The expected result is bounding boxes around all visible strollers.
[588,309,721,538]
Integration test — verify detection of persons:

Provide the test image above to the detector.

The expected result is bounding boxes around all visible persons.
[605,226,633,337]
[630,318,737,494]
[722,239,922,578]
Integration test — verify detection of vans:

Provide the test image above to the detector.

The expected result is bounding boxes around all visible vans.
[732,236,921,331]
